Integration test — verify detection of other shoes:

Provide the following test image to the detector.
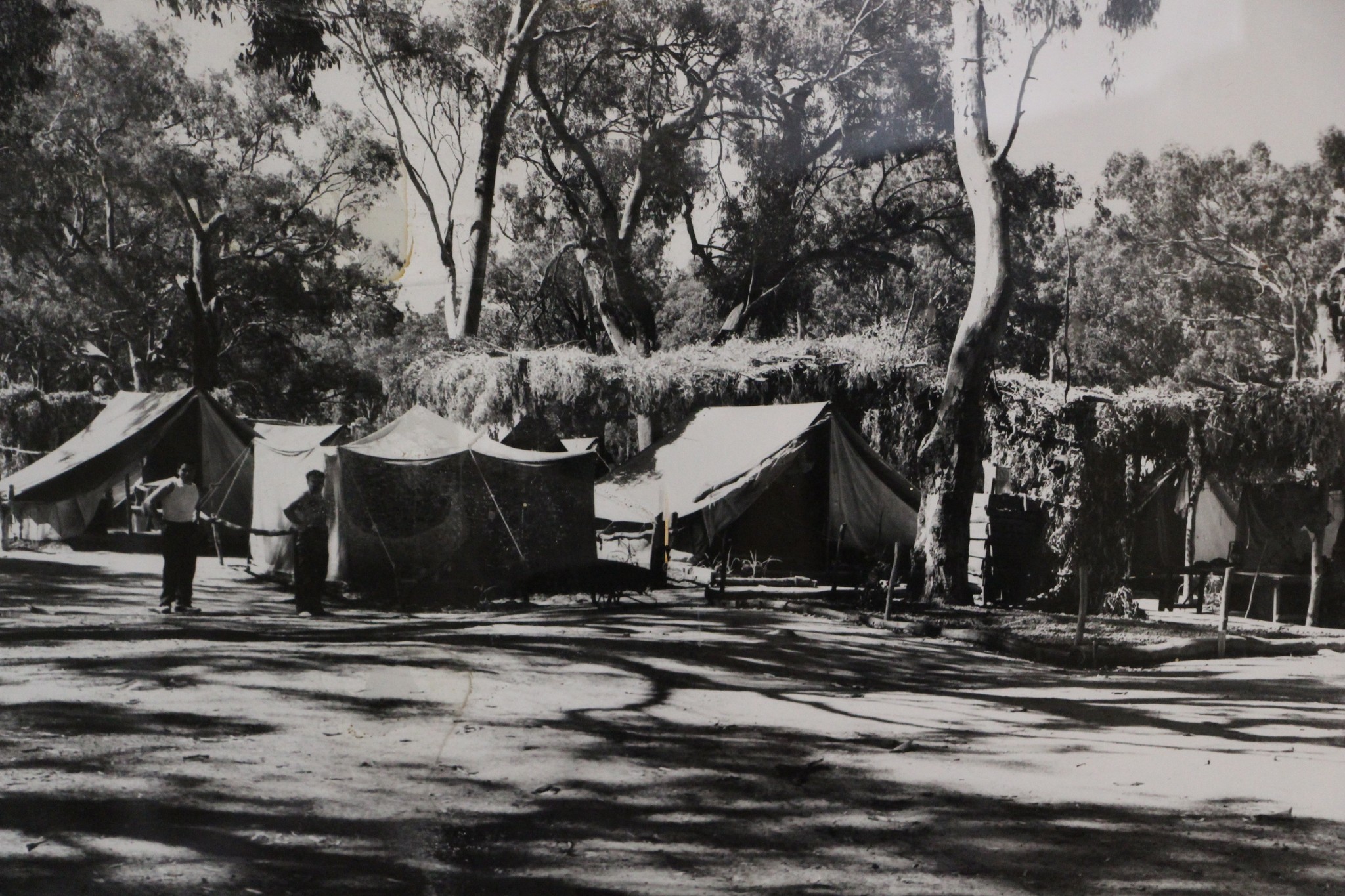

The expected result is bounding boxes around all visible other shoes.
[298,611,311,617]
[174,605,201,615]
[316,610,334,617]
[159,605,171,613]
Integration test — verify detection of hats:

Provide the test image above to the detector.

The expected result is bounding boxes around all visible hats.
[306,470,325,483]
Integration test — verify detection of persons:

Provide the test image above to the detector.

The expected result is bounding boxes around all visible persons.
[284,470,330,616]
[147,456,202,615]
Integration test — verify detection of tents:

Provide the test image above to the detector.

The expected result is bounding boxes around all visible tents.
[593,401,920,600]
[2,386,265,556]
[254,405,599,598]
